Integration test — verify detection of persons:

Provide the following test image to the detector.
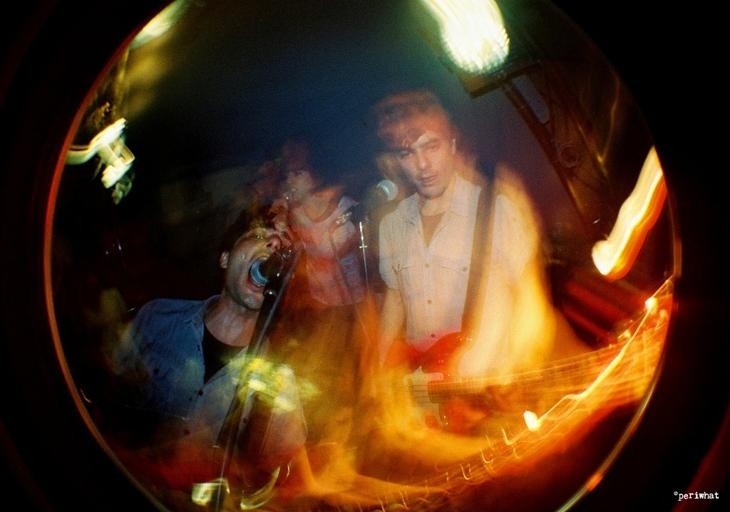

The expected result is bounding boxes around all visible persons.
[48,89,672,511]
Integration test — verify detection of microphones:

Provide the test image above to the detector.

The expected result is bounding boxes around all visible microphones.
[247,247,292,288]
[335,176,400,228]
[268,189,295,226]
